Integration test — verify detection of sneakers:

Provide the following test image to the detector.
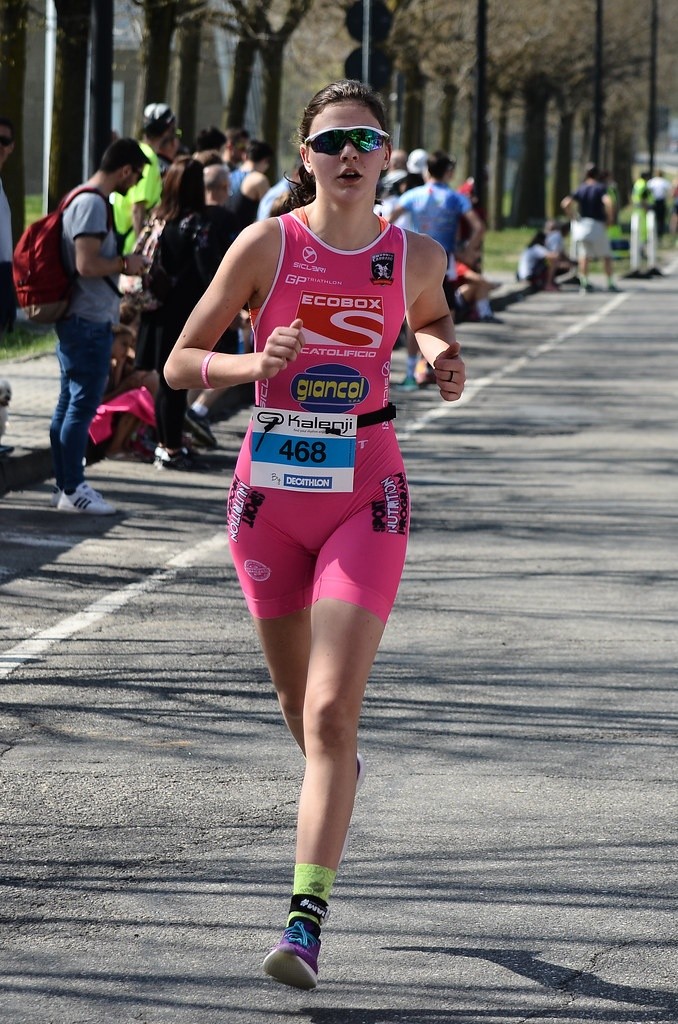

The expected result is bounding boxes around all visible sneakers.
[392,377,420,393]
[56,488,116,515]
[353,751,367,796]
[49,483,104,507]
[102,408,215,472]
[262,920,322,989]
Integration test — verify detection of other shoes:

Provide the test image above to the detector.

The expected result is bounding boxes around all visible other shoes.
[579,283,599,293]
[469,313,505,325]
[413,372,438,384]
[604,284,623,292]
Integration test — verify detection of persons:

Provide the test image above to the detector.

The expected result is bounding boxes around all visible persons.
[372,149,502,391]
[89,103,272,470]
[516,218,596,293]
[603,170,678,249]
[50,138,146,513]
[560,163,624,293]
[256,154,316,220]
[0,116,18,338]
[164,82,466,991]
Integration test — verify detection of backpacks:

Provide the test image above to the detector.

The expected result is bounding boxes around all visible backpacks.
[10,184,114,327]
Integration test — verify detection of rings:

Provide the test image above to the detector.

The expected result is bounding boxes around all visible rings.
[447,371,453,382]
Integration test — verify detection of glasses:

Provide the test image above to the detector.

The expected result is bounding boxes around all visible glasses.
[232,143,251,153]
[302,126,391,157]
[134,169,143,185]
[0,135,14,146]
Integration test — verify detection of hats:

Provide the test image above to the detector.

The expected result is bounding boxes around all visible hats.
[141,102,177,135]
[378,169,412,193]
[405,149,430,175]
[455,177,475,199]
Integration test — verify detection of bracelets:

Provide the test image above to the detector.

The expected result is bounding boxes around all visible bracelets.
[121,256,129,274]
[201,352,217,389]
